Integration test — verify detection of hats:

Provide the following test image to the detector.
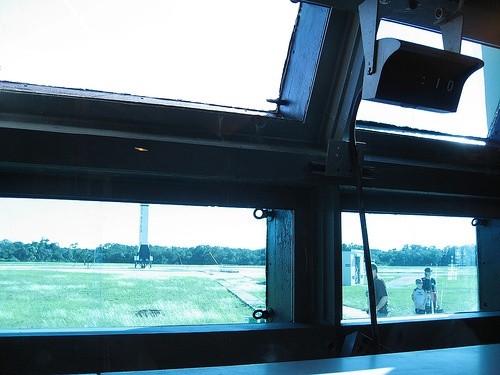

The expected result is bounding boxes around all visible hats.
[416,279,423,284]
[425,268,431,272]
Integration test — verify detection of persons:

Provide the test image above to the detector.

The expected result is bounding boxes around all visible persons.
[421,267,436,314]
[366,262,388,318]
[411,278,427,314]
